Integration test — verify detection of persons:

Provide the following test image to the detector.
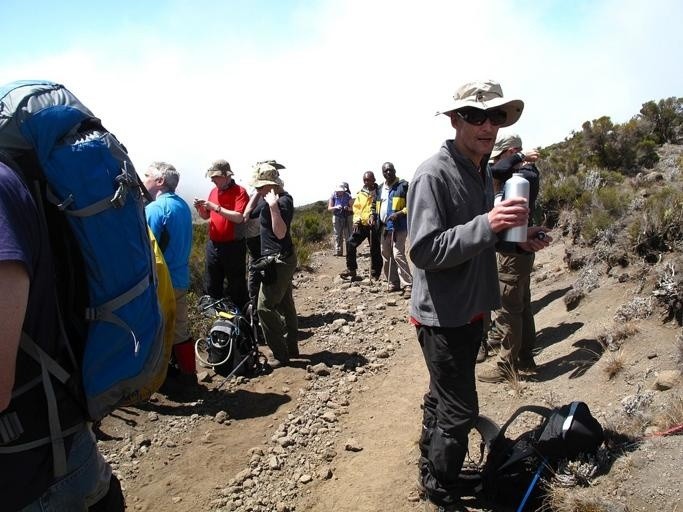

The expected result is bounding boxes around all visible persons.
[478,137,540,383]
[367,162,413,299]
[145,164,198,386]
[0,163,124,512]
[474,157,504,363]
[242,160,285,323]
[405,82,553,512]
[327,186,357,256]
[242,168,299,369]
[194,163,250,325]
[339,172,383,281]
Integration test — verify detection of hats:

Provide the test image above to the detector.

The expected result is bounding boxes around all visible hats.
[336,183,347,192]
[490,133,522,159]
[436,81,524,127]
[253,159,285,188]
[207,160,233,178]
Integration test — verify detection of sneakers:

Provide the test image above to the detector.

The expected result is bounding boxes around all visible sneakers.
[404,287,411,299]
[417,471,482,497]
[424,498,469,512]
[261,349,299,368]
[338,269,356,277]
[384,284,400,292]
[477,338,536,383]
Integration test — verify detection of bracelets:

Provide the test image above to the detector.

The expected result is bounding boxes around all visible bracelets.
[517,151,527,163]
[215,205,221,213]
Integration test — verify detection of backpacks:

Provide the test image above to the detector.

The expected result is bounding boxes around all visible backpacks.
[208,311,258,377]
[483,401,602,505]
[0,81,177,455]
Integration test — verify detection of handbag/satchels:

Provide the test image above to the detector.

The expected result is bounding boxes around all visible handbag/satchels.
[251,257,277,282]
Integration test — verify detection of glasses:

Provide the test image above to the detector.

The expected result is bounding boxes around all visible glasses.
[457,109,507,125]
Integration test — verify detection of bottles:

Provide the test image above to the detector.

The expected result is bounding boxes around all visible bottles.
[500,172,529,244]
